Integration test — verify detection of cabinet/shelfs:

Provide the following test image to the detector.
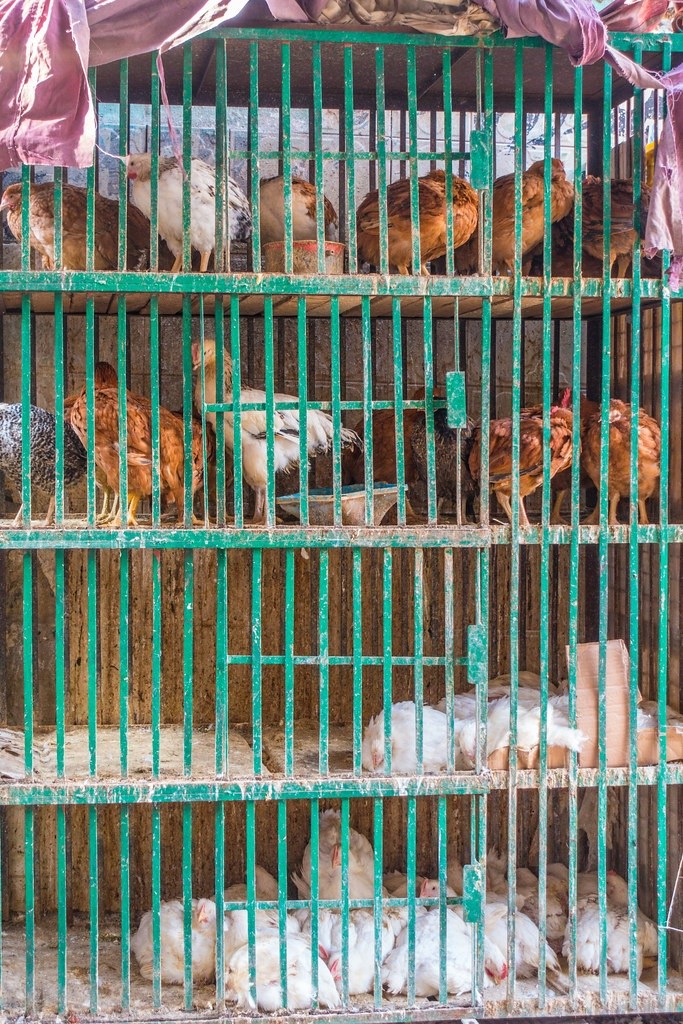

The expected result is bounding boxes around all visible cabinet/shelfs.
[0,0,681,1023]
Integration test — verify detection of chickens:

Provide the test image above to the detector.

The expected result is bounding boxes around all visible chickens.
[63,361,216,528]
[409,408,480,525]
[345,169,481,275]
[0,181,150,270]
[131,808,656,1012]
[0,401,98,531]
[248,174,339,245]
[361,670,683,778]
[127,153,252,273]
[342,385,442,525]
[429,156,574,277]
[189,338,366,525]
[557,174,652,278]
[466,386,661,526]
